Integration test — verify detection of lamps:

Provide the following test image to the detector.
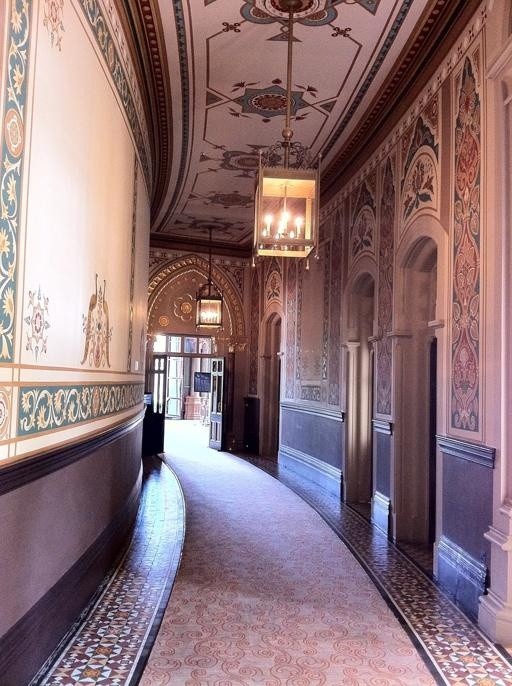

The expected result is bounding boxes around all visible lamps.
[194,224,224,333]
[250,0,322,272]
